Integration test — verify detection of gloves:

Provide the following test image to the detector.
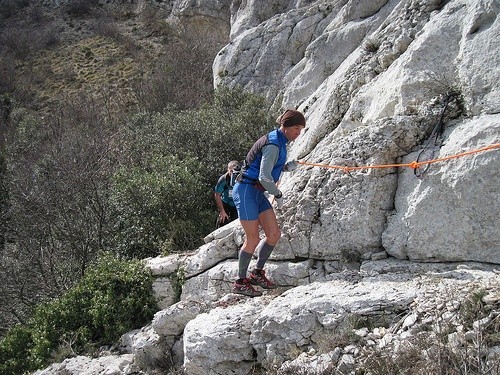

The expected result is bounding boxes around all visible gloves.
[281,159,298,172]
[274,190,283,208]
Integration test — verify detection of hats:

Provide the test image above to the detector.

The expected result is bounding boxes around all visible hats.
[276,109,306,128]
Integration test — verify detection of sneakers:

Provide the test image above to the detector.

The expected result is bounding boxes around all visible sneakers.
[248,270,276,289]
[231,277,263,296]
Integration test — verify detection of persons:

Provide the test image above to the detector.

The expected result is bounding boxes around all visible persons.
[214,160,241,229]
[233,109,306,297]
